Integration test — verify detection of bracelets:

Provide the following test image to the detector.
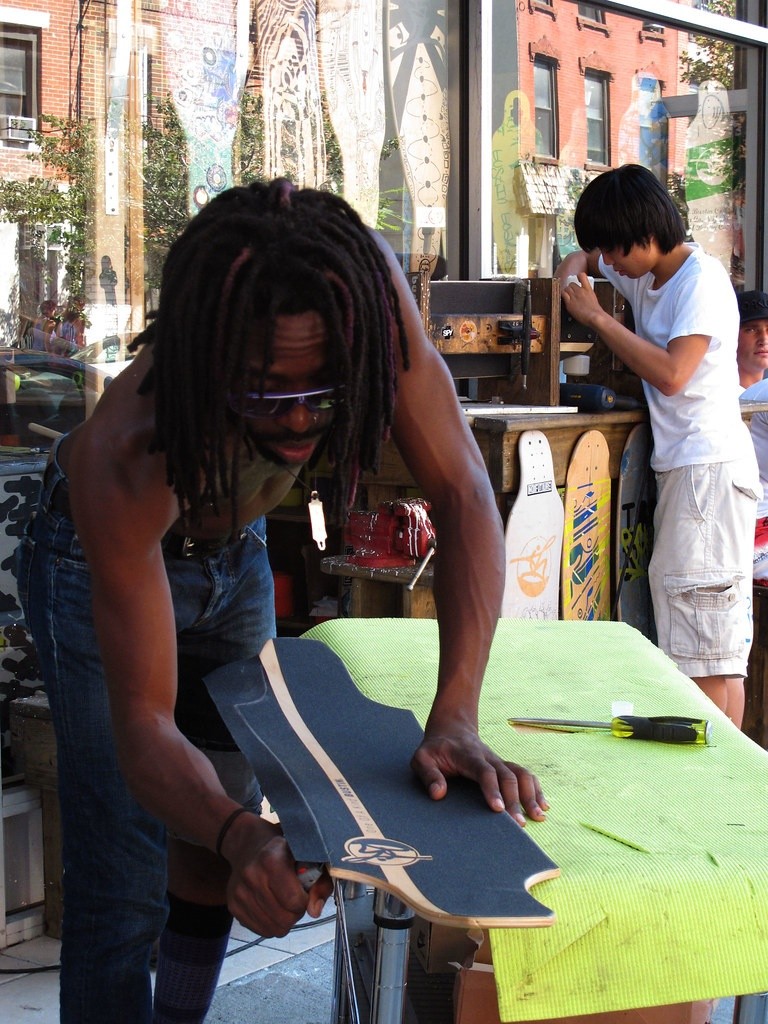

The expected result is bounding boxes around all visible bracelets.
[216,805,262,852]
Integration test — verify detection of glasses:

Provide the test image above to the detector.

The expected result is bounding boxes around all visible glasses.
[225,381,346,421]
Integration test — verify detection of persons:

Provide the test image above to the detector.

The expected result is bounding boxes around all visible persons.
[31,294,93,357]
[736,289,768,752]
[551,163,763,736]
[16,178,548,1024]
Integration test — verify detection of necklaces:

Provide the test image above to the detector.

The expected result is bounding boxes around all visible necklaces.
[278,461,329,551]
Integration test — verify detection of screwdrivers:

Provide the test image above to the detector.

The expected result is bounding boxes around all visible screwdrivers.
[508,714,711,746]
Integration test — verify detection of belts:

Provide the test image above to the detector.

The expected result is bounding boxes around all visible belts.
[44,463,232,559]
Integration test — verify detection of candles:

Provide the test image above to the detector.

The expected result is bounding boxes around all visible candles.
[516,232,529,279]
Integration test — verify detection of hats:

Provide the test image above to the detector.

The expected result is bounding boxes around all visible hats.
[736,290,768,323]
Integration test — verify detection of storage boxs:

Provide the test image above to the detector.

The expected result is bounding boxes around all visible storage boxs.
[414,909,718,1022]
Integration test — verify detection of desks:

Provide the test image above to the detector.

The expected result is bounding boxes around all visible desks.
[299,613,768,1024]
[269,398,768,635]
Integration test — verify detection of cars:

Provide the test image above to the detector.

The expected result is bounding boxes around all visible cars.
[69,333,146,379]
[0,309,40,349]
[0,347,117,450]
